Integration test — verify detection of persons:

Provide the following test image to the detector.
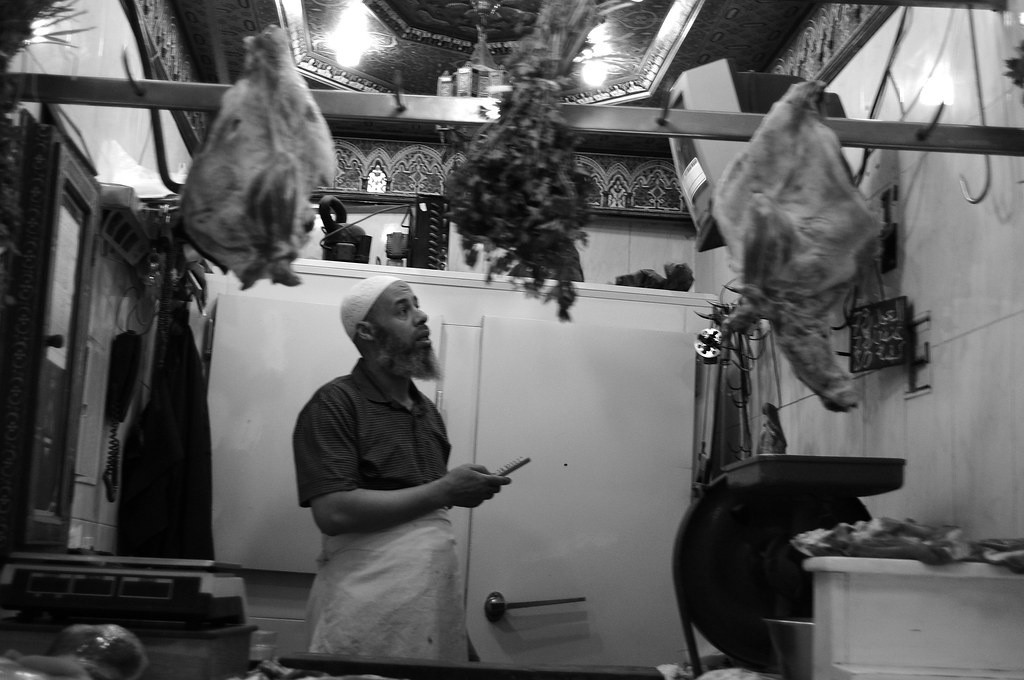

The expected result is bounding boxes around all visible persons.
[292,276,512,680]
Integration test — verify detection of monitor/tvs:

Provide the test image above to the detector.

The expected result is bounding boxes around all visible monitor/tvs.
[669,57,847,232]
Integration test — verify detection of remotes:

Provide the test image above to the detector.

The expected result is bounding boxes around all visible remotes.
[492,456,531,476]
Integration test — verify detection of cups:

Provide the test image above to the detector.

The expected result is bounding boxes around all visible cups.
[354,234,372,263]
[337,243,352,261]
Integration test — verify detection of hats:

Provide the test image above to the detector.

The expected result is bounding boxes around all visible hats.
[341,276,401,341]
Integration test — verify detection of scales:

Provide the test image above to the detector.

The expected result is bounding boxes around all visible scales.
[1,553,259,641]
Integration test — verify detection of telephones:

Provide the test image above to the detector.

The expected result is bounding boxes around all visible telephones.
[105,331,142,424]
